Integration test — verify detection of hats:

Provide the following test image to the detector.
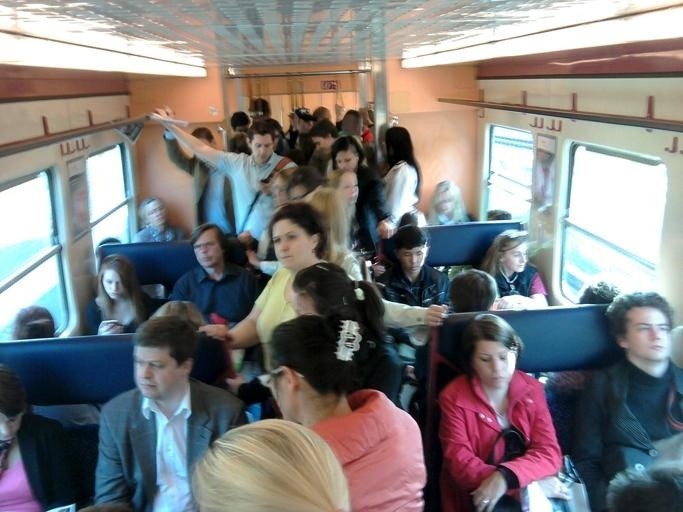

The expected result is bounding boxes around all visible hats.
[293,107,317,123]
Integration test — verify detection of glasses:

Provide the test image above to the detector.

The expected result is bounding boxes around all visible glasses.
[190,239,222,253]
[258,364,305,391]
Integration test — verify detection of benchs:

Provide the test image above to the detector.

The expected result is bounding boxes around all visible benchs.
[433,297,613,373]
[0,332,224,505]
[96,233,248,313]
[414,220,521,271]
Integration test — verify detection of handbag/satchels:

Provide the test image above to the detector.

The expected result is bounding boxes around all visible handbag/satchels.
[484,428,592,512]
